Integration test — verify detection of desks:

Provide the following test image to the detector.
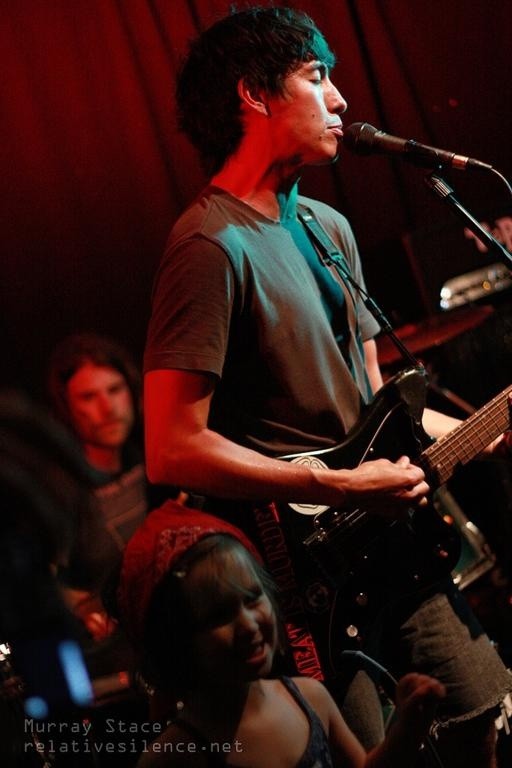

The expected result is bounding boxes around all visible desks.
[377,304,493,418]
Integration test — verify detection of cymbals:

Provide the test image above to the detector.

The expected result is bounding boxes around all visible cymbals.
[374,304,493,365]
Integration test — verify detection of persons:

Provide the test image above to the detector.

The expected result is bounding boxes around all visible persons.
[42,326,198,644]
[112,496,447,768]
[138,4,511,766]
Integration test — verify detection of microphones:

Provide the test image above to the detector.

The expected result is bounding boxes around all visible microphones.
[344,120,491,176]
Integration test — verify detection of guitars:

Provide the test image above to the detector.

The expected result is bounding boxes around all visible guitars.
[274,365,512,647]
